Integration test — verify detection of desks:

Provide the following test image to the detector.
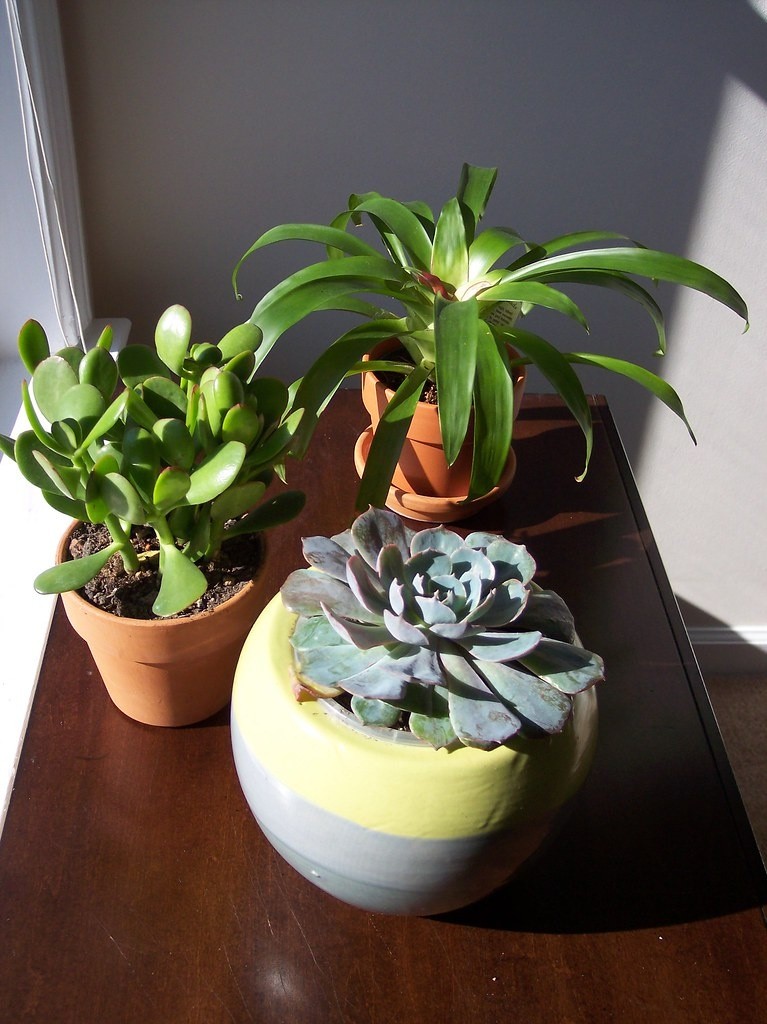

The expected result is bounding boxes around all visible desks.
[0,391,767,1024]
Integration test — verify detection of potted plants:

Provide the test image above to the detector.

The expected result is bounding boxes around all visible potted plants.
[0,302,307,725]
[232,163,749,523]
[229,501,604,915]
